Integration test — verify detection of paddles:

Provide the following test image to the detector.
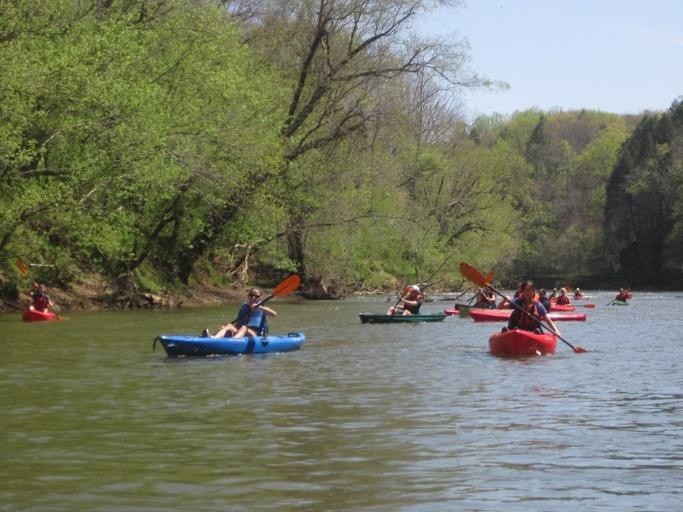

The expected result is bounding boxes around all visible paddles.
[231,275,301,323]
[15,257,67,322]
[566,304,596,308]
[392,288,413,315]
[468,270,496,309]
[457,261,587,352]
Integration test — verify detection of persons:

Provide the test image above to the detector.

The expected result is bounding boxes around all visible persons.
[386,283,424,317]
[28,282,52,313]
[497,279,560,337]
[201,285,276,339]
[472,280,633,311]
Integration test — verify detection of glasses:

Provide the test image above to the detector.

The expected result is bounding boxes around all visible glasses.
[249,293,260,298]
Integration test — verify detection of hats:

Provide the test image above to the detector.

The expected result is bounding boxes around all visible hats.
[409,285,419,293]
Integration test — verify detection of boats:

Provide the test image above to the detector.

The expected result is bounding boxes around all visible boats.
[485,327,561,357]
[18,307,57,324]
[153,328,307,359]
[444,284,632,322]
[353,304,448,325]
